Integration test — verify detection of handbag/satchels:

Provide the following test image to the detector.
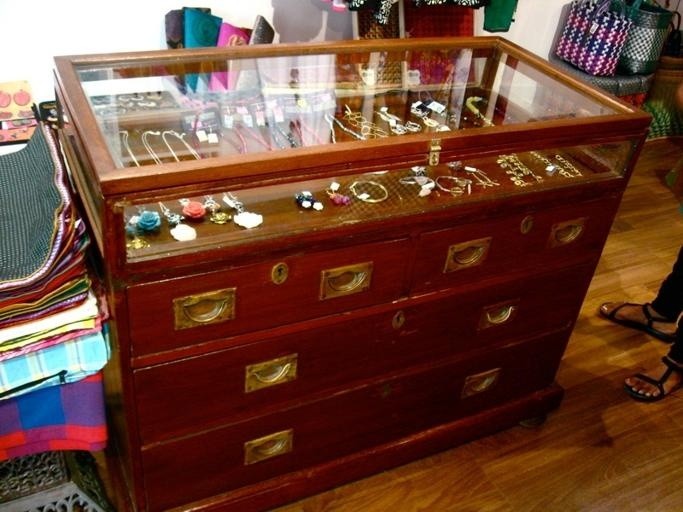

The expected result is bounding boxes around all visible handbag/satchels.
[556,0,633,77]
[597,0,673,74]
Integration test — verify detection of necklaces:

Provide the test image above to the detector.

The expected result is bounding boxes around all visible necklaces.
[107,95,276,251]
[268,96,581,213]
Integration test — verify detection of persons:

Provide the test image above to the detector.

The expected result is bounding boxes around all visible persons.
[598,244,682,403]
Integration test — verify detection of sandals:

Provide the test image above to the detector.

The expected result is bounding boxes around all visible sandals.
[624,356,683,402]
[600,302,679,343]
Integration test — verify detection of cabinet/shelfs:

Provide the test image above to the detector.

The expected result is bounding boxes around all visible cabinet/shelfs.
[49,36,653,511]
